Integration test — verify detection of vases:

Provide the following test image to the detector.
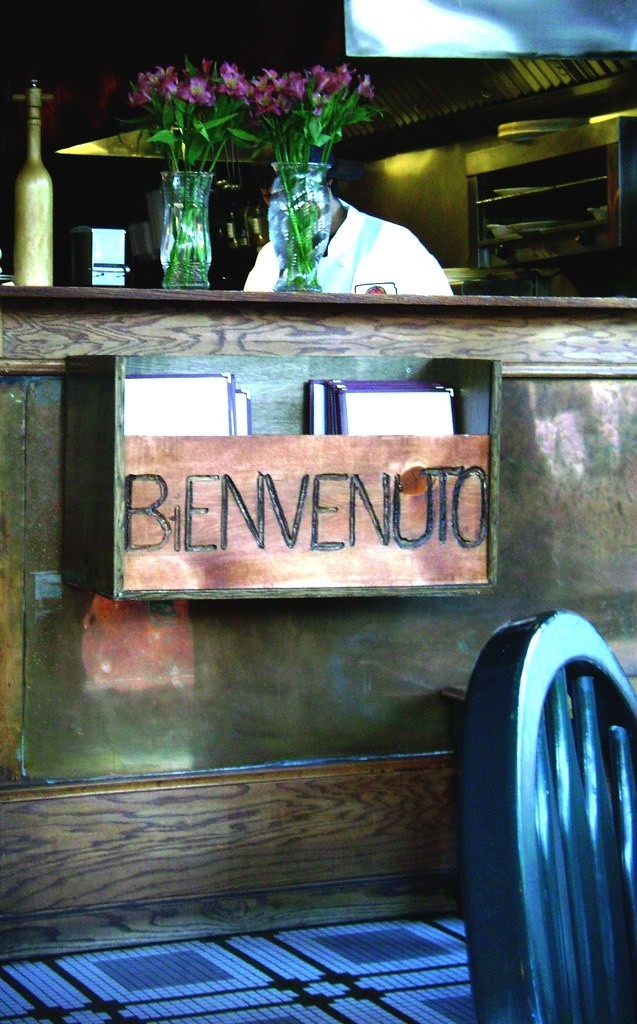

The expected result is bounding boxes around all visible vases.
[161,172,213,289]
[264,161,332,292]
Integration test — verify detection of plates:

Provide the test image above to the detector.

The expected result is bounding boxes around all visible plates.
[507,221,565,233]
[492,187,539,196]
[497,117,589,143]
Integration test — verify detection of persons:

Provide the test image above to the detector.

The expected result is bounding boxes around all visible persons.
[242,144,452,297]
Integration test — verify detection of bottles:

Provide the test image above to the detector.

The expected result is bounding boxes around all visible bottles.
[14,80,54,285]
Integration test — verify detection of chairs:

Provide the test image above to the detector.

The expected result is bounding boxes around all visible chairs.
[460,608,637,1024]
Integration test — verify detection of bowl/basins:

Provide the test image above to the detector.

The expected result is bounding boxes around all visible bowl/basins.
[487,224,511,237]
[587,208,607,220]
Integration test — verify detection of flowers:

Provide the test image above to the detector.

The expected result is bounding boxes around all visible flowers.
[114,62,390,288]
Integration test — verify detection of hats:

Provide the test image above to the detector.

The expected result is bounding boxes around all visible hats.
[245,144,337,189]
